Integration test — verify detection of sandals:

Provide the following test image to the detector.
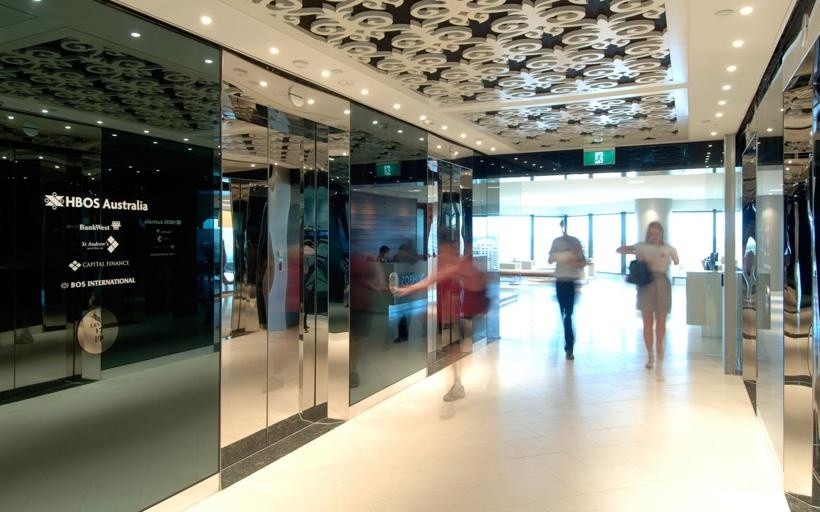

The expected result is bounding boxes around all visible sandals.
[655,362,665,382]
[645,352,655,368]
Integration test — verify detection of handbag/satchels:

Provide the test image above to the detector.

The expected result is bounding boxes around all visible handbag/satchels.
[626,260,651,285]
[556,259,585,281]
[463,289,490,317]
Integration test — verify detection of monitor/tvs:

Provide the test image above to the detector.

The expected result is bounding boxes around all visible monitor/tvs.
[710,252,715,271]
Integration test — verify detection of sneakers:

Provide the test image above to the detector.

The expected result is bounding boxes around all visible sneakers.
[350,372,358,388]
[443,384,465,400]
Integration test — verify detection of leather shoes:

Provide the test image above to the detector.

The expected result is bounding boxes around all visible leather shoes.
[564,343,574,360]
[393,335,408,343]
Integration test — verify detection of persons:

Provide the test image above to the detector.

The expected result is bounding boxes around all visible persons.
[351,229,389,390]
[391,227,490,403]
[377,246,390,262]
[392,240,418,343]
[617,222,679,372]
[548,220,587,360]
